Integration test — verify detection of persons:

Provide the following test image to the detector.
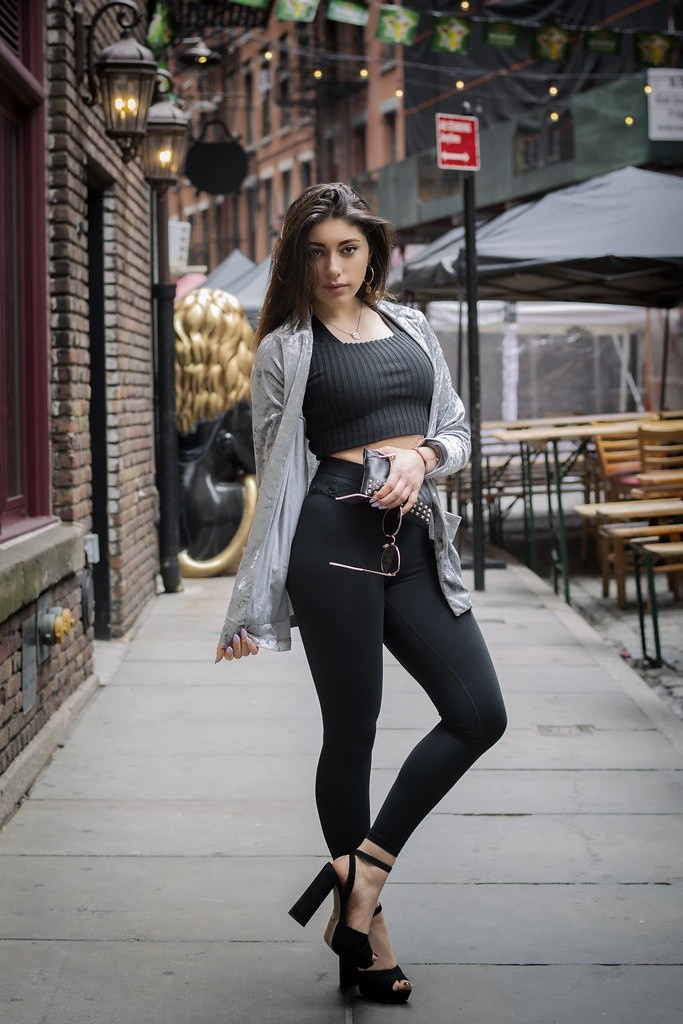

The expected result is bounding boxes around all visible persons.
[215,181,509,1004]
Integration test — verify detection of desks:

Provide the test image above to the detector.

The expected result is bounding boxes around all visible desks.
[492,421,683,607]
[479,408,683,575]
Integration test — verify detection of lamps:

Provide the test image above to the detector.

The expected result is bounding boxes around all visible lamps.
[172,36,222,67]
[137,68,193,195]
[72,0,158,165]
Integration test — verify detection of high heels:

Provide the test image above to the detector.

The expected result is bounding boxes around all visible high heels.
[339,903,412,1003]
[288,850,392,969]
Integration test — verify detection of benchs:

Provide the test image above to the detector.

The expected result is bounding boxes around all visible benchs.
[434,414,683,670]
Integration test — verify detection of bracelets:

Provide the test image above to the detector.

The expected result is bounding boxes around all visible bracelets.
[412,448,428,476]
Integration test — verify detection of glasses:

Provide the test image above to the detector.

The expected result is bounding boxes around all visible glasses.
[329,494,402,575]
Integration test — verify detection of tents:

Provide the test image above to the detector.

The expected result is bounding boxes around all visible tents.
[200,168,683,599]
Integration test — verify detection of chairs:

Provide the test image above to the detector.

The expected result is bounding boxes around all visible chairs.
[631,421,683,528]
[592,419,676,611]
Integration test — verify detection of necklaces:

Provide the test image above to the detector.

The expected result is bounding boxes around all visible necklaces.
[311,299,365,345]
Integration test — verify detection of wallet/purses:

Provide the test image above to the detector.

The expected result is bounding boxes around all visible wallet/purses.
[361,447,433,527]
